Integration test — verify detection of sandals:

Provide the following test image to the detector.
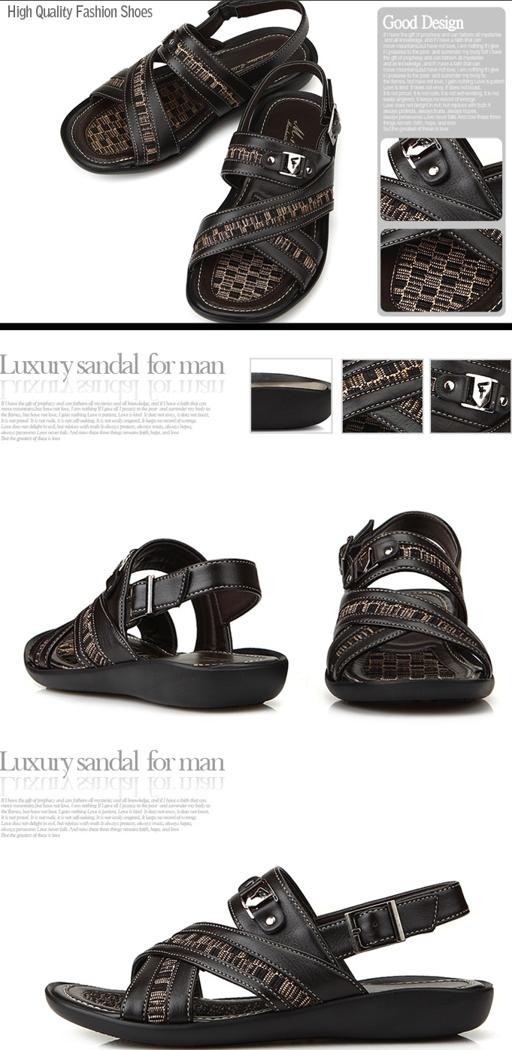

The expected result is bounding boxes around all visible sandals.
[60,0,319,174]
[430,359,511,433]
[380,137,503,222]
[185,59,341,324]
[380,228,502,313]
[23,539,288,709]
[251,372,332,431]
[342,359,423,433]
[325,511,494,704]
[44,866,494,1047]
